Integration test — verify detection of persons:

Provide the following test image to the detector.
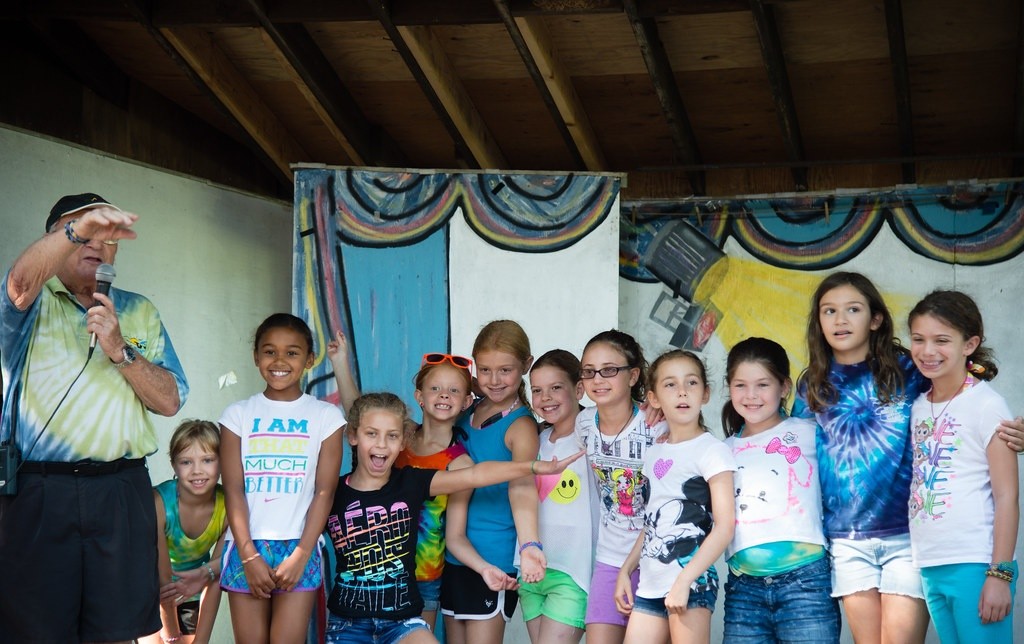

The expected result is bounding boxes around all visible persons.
[614,336,842,644]
[439,320,547,644]
[790,271,1024,644]
[517,329,665,644]
[158,311,586,644]
[0,192,189,644]
[133,417,231,644]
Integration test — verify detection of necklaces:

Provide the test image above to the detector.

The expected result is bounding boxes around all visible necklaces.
[931,377,967,431]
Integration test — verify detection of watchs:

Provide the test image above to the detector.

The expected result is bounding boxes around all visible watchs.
[108,343,137,369]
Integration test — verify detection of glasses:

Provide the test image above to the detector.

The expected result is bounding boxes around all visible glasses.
[420,352,473,379]
[104,240,118,244]
[578,364,632,378]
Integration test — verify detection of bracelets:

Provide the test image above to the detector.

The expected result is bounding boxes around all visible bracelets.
[202,561,215,581]
[519,540,544,554]
[241,553,260,564]
[531,461,537,475]
[985,564,1015,581]
[160,628,183,642]
[65,218,90,243]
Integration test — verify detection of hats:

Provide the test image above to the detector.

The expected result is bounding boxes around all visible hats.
[44,193,121,234]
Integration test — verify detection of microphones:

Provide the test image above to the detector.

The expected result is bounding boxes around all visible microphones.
[87,263,116,359]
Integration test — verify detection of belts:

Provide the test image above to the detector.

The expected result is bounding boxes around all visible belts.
[21,457,146,476]
